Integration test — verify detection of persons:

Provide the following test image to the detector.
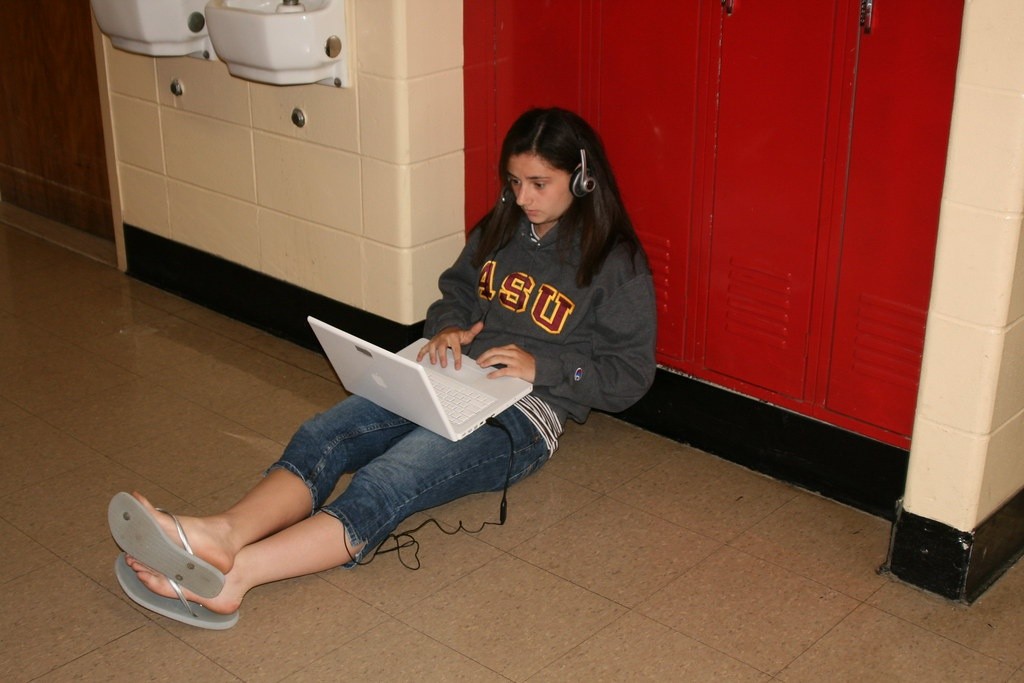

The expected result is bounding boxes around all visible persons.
[107,107,656,630]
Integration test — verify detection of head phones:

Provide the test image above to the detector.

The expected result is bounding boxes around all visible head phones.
[569,148,597,199]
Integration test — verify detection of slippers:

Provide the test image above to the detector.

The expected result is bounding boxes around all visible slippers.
[114,552,239,630]
[107,493,225,598]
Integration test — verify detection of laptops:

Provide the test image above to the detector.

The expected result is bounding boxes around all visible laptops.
[307,316,533,442]
